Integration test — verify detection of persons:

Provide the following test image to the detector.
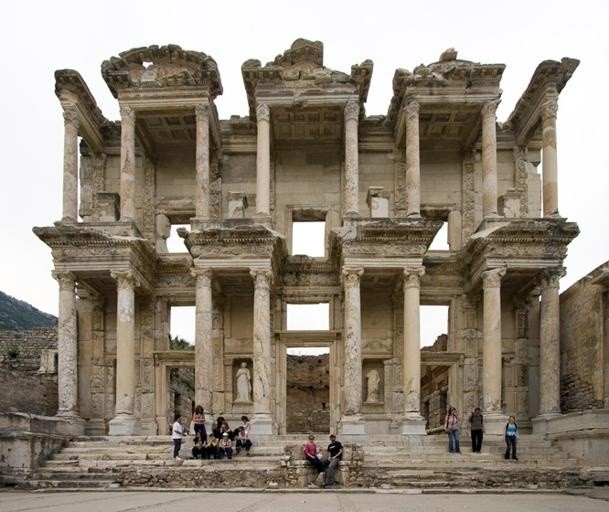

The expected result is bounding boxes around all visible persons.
[502,414,521,461]
[235,360,252,403]
[171,403,253,462]
[325,434,344,487]
[303,434,325,475]
[466,406,487,454]
[366,368,382,403]
[444,406,462,454]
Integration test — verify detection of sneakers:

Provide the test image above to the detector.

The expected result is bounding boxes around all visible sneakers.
[504,456,518,460]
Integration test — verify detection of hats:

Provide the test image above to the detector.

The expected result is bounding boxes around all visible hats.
[223,433,228,436]
[330,435,336,438]
